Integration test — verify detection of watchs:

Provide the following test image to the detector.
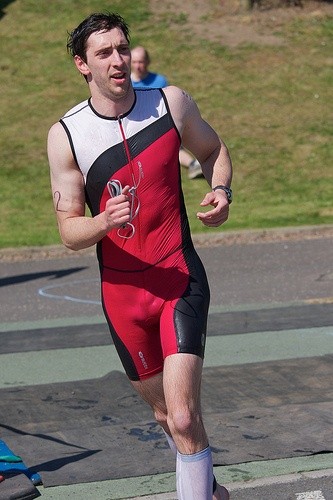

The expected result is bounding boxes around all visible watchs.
[212,185,233,204]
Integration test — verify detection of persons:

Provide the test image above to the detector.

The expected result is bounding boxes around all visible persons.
[47,10,234,500]
[129,46,203,179]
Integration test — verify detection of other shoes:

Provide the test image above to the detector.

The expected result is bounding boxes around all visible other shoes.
[188,164,206,178]
